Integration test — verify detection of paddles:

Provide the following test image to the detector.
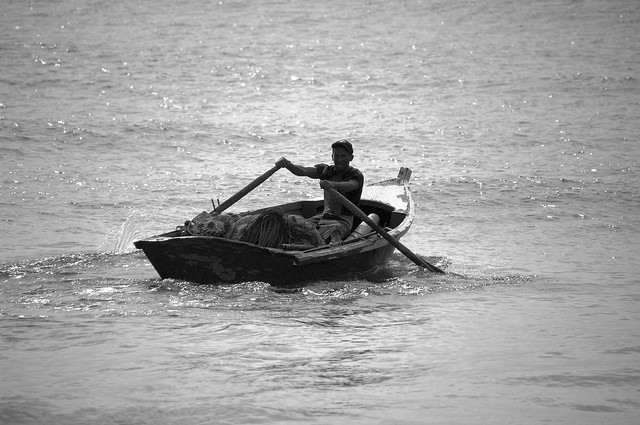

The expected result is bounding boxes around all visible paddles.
[326,187,445,274]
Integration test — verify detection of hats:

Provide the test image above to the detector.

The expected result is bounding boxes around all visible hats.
[332,138,353,152]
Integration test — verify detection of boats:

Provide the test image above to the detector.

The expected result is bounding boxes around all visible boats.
[133,166,413,289]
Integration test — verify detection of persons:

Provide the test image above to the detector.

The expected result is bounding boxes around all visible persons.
[275,139,364,245]
